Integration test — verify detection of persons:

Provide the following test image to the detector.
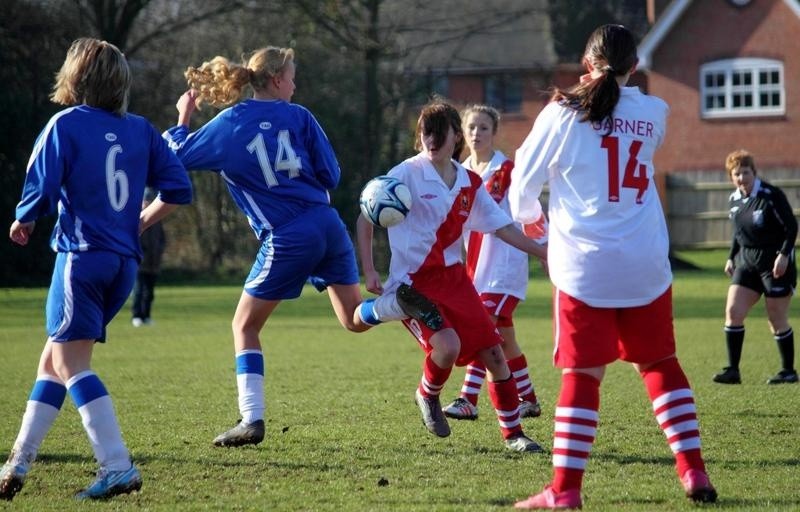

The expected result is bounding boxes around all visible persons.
[439,105,548,420]
[356,102,547,453]
[712,146,798,386]
[513,26,717,511]
[129,193,163,327]
[1,36,194,505]
[159,47,442,449]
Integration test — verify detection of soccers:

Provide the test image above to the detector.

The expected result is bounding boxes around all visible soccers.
[360,175,411,227]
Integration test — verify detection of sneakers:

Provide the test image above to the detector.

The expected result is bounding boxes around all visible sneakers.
[713,364,740,384]
[414,386,545,456]
[683,469,717,502]
[515,484,582,509]
[76,459,141,497]
[213,419,265,446]
[1,463,27,500]
[769,369,798,384]
[396,281,442,330]
